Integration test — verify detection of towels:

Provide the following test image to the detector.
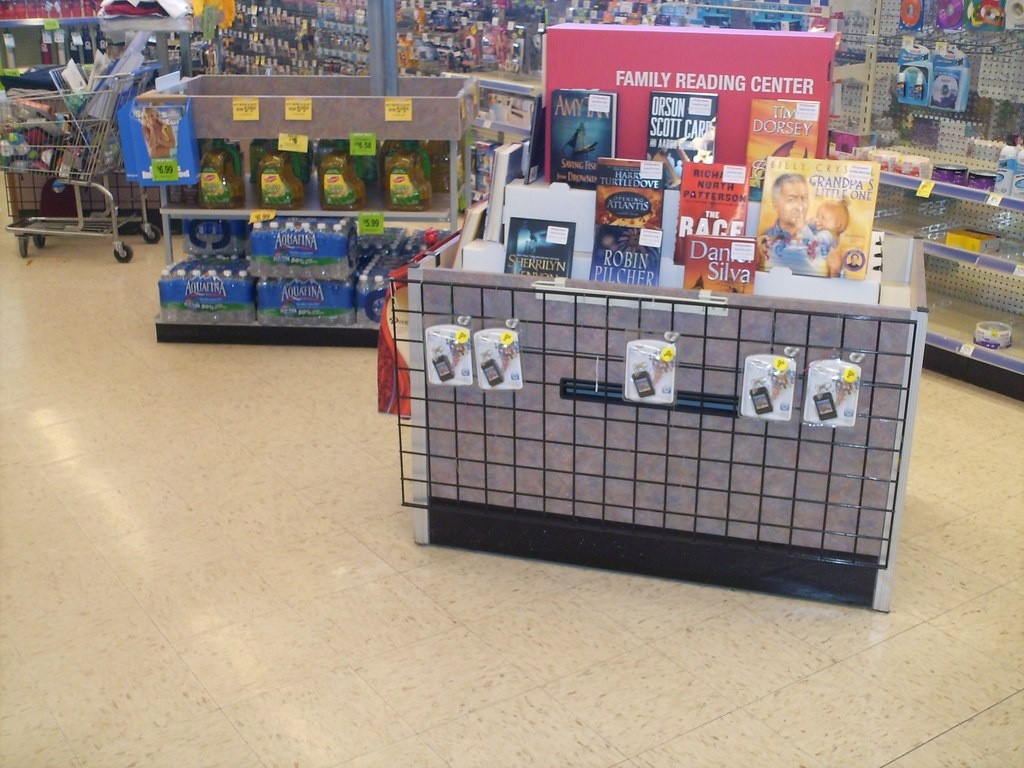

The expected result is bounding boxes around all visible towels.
[95,1,195,20]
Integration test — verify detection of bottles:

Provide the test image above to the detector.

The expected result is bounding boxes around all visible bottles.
[315,138,378,209]
[3,28,16,69]
[994,134,1024,201]
[157,218,451,327]
[248,138,311,211]
[40,24,106,65]
[183,139,246,208]
[380,140,466,211]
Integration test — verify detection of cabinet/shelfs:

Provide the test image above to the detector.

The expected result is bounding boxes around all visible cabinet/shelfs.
[475,0,1024,375]
[135,75,478,348]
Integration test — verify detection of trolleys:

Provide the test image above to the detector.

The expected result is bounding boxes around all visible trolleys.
[0,60,163,263]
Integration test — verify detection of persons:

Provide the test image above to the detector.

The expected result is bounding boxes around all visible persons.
[142,108,176,156]
[848,254,860,268]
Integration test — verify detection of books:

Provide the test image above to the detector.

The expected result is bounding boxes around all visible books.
[594,157,665,229]
[550,88,619,190]
[589,223,663,286]
[673,162,752,265]
[745,99,820,202]
[647,91,718,189]
[684,235,757,294]
[757,157,882,281]
[505,217,576,277]
